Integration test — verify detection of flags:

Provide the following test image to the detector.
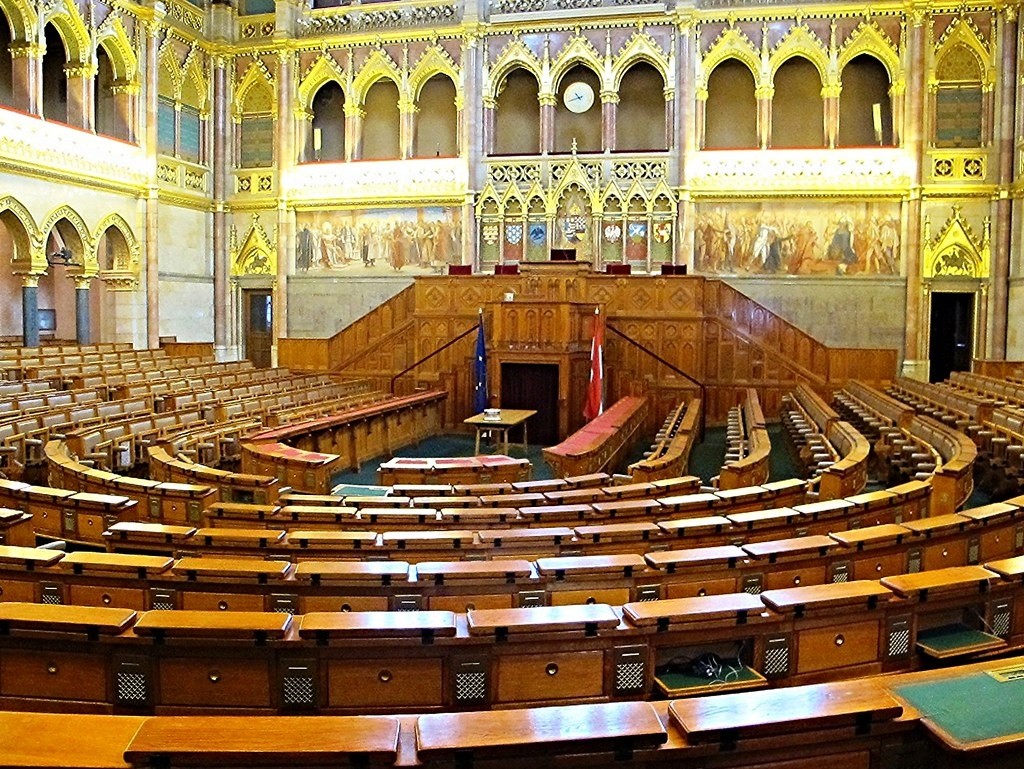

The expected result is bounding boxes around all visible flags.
[583,313,603,420]
[474,314,488,414]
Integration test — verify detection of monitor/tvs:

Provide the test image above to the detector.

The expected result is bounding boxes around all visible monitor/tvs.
[550,249,577,260]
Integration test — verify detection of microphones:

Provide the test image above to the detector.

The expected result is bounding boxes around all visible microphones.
[501,262,505,275]
